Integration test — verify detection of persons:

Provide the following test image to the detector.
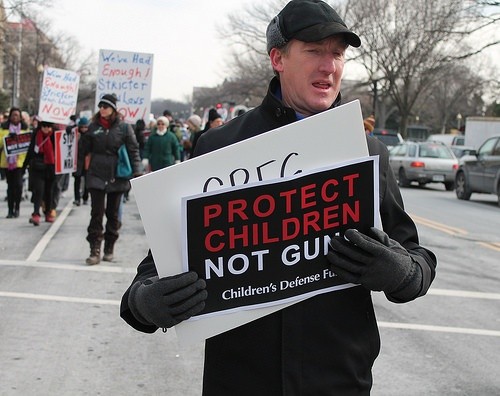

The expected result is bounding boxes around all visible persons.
[121,1,437,395]
[67,94,142,265]
[0,101,247,225]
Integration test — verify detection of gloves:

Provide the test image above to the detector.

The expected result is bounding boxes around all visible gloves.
[326,227,423,304]
[128,271,208,333]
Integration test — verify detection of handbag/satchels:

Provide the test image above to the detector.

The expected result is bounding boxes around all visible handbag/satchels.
[116,122,132,179]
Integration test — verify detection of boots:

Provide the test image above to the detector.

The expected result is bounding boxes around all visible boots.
[6,198,20,218]
[86,240,101,264]
[103,236,115,261]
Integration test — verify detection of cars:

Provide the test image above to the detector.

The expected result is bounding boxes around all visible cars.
[389,140,500,202]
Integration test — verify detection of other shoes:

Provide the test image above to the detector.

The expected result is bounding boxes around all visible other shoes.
[73,201,80,206]
[29,212,40,226]
[45,211,54,222]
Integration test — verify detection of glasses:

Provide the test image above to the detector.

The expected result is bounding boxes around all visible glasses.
[98,104,110,109]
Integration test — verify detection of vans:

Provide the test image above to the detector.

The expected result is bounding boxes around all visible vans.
[372,129,405,153]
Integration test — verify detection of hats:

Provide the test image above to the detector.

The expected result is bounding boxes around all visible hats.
[208,108,221,121]
[78,117,90,127]
[363,112,376,132]
[163,110,171,115]
[266,0,361,56]
[189,114,201,128]
[156,116,169,128]
[100,93,118,110]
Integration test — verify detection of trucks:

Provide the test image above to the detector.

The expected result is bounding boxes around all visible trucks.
[428,116,500,154]
